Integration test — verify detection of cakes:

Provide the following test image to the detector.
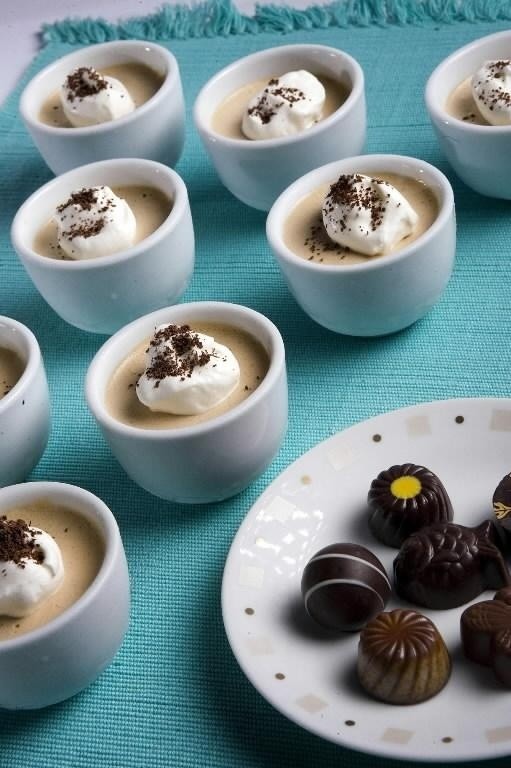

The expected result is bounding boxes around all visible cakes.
[359,607,450,705]
[369,463,452,551]
[493,470,511,528]
[393,521,505,608]
[301,543,390,632]
[459,585,510,686]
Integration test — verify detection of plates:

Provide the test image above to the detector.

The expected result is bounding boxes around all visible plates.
[220,397,511,762]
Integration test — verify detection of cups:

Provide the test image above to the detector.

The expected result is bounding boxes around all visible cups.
[193,43,367,214]
[426,29,510,200]
[84,301,290,506]
[18,40,187,176]
[11,157,194,336]
[266,154,458,338]
[0,481,130,712]
[1,312,53,487]
